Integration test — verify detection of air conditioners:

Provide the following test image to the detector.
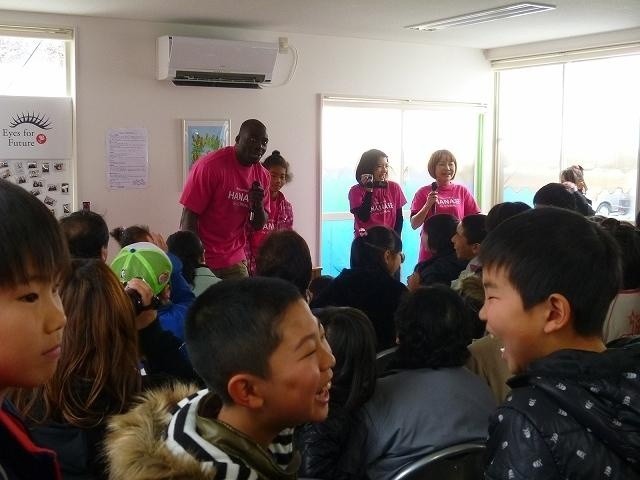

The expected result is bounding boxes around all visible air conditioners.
[157,35,279,93]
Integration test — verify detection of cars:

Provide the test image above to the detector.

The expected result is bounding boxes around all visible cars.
[577,172,636,219]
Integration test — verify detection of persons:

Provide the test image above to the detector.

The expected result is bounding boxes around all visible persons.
[306,307,378,478]
[308,276,334,299]
[406,214,458,286]
[110,225,158,247]
[344,286,502,480]
[111,243,197,376]
[60,211,108,261]
[320,225,408,306]
[406,263,441,289]
[410,150,479,263]
[5,258,195,426]
[451,214,488,261]
[348,149,407,279]
[254,229,312,294]
[178,119,270,277]
[478,164,640,480]
[0,177,68,480]
[245,150,293,275]
[166,231,223,297]
[98,276,336,480]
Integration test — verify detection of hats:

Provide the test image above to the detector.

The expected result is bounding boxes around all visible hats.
[109,241,173,295]
[166,229,204,254]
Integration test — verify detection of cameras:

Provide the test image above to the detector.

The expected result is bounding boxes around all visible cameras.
[125,278,161,311]
[361,173,375,185]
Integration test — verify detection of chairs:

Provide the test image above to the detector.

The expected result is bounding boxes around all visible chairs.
[391,442,486,480]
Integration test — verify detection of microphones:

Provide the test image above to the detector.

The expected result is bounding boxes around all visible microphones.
[430,181,441,215]
[247,179,263,224]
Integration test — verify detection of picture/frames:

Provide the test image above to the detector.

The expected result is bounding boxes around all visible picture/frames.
[182,119,231,190]
